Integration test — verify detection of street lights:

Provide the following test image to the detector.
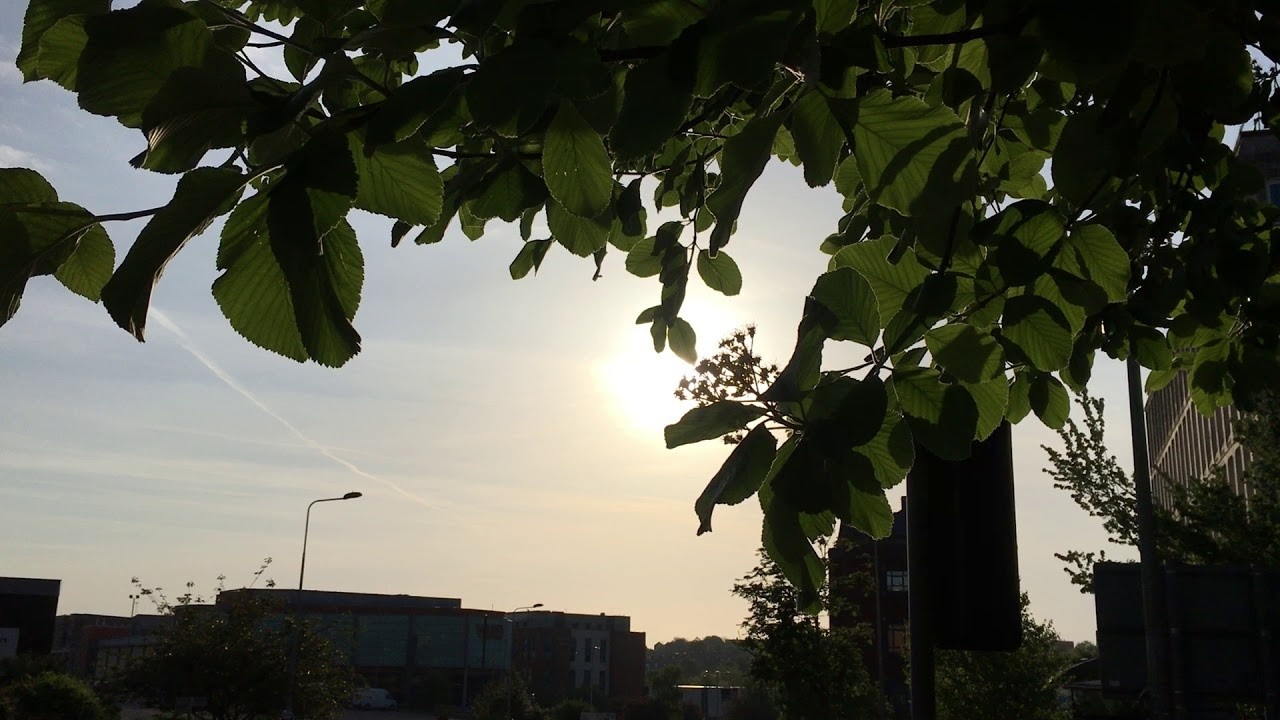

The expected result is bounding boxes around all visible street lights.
[130,594,140,617]
[298,490,363,591]
[506,603,544,720]
[716,670,720,720]
